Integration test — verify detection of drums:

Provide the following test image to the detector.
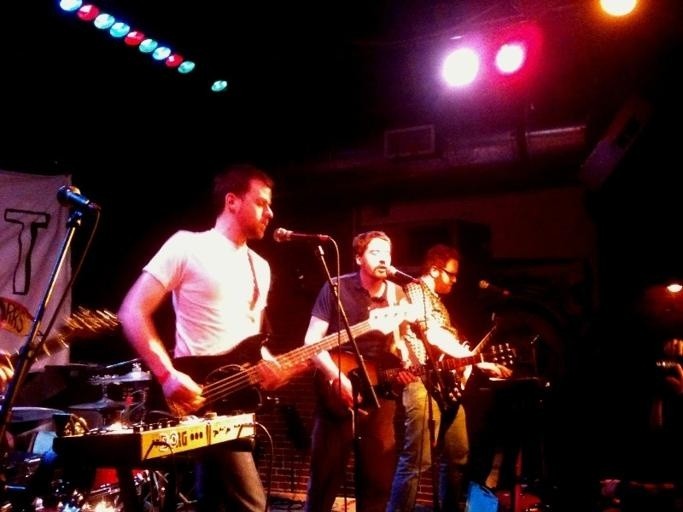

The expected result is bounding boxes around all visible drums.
[85,467,151,497]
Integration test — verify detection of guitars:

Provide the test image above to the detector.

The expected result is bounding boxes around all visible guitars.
[313,342,519,423]
[145,300,422,471]
[0,306,121,371]
[424,314,506,409]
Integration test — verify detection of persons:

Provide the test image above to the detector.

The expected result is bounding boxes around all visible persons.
[386,244,512,512]
[0,364,15,385]
[117,166,282,512]
[304,230,421,512]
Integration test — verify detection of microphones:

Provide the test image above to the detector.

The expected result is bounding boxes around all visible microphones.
[273,228,330,244]
[386,265,419,284]
[57,185,101,213]
[479,280,510,297]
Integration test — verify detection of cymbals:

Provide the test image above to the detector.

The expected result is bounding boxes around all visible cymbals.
[68,399,139,410]
[103,371,152,382]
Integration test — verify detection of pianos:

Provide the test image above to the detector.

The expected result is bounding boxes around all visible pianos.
[52,411,256,465]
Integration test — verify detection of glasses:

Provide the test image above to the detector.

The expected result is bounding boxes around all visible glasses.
[436,264,458,279]
